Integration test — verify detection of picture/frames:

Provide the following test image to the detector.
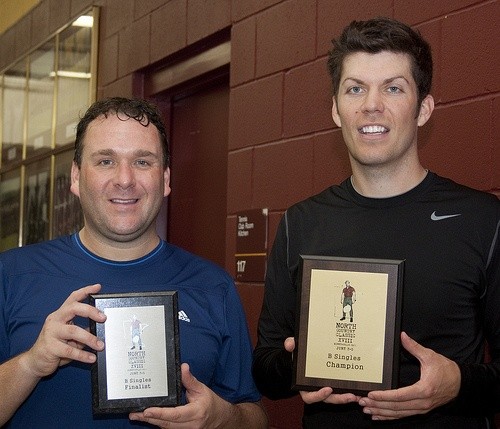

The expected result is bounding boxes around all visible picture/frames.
[88,291,182,421]
[290,253,407,397]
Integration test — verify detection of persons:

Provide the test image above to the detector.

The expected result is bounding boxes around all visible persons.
[250,17,500,429]
[0,96,269,429]
